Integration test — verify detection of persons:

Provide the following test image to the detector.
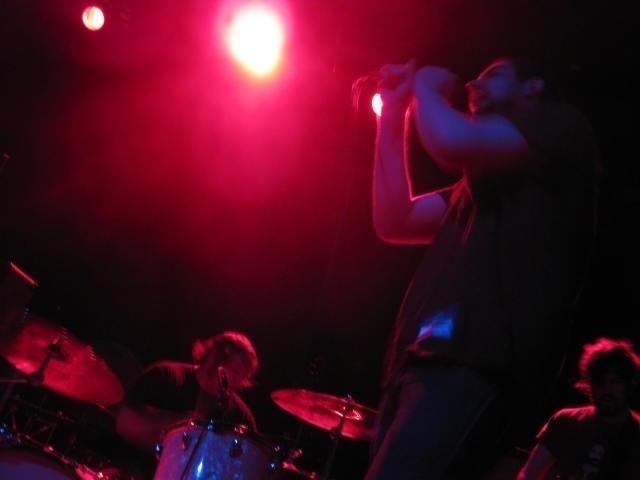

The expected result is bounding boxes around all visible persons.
[363,52,605,480]
[515,336,640,480]
[113,330,261,470]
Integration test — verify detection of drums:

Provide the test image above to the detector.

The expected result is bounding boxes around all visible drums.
[154,420,278,479]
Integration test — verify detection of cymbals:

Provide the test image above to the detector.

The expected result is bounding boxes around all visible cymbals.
[272,390,383,441]
[4,322,122,408]
